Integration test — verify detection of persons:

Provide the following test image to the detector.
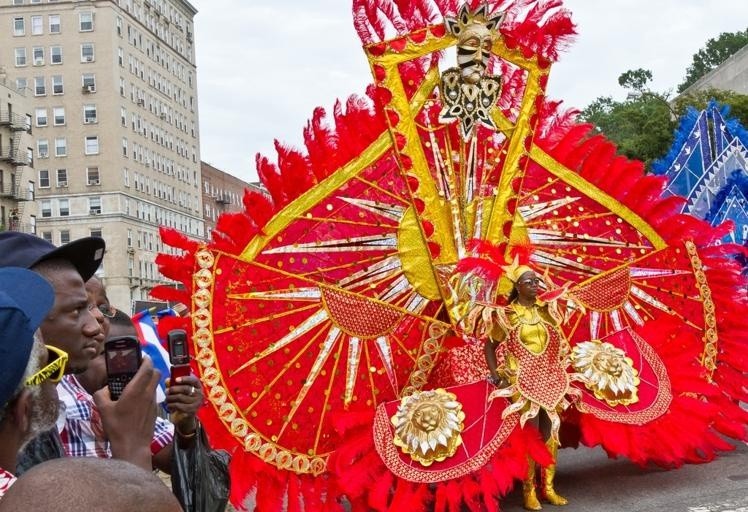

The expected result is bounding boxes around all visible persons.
[1,229,230,511]
[484,263,568,510]
[456,24,493,86]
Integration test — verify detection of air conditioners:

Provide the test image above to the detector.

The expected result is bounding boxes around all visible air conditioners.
[159,112,167,118]
[86,54,95,93]
[137,97,146,105]
[90,179,101,215]
[186,31,192,37]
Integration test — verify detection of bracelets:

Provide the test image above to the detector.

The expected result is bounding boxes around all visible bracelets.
[493,378,500,385]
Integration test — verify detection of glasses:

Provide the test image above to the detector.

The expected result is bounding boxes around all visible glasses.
[89,304,117,318]
[518,278,539,285]
[5,344,69,405]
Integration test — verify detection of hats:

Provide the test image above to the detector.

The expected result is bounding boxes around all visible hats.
[0,230,105,283]
[510,264,533,284]
[0,267,54,406]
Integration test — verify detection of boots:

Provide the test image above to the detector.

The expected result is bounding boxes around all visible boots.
[522,454,542,510]
[541,438,568,505]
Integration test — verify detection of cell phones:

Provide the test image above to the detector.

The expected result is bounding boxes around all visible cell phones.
[104,335,143,400]
[167,329,192,403]
